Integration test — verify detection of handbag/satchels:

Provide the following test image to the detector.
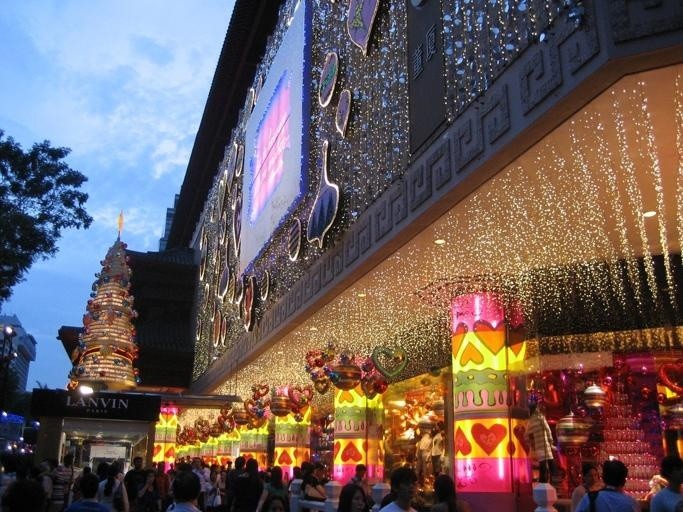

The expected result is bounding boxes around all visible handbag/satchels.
[112,481,126,512]
[304,483,327,502]
[213,488,222,507]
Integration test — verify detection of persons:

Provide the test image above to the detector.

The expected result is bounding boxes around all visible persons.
[574,460,642,512]
[650,455,683,512]
[570,462,605,512]
